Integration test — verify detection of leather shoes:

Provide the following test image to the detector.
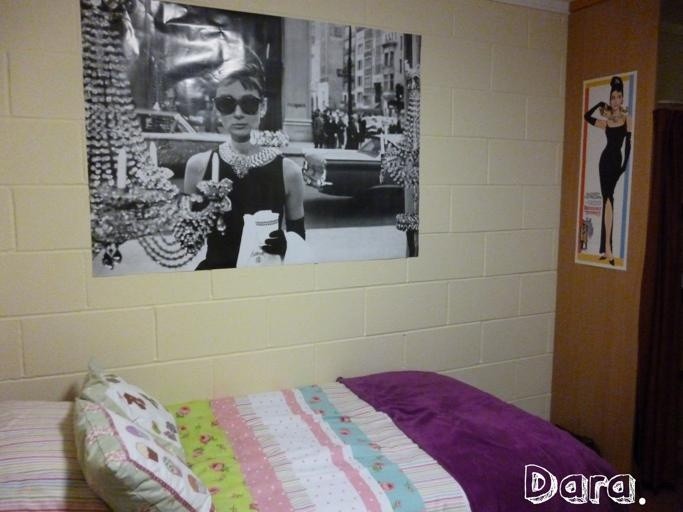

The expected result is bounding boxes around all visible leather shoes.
[599,254,615,266]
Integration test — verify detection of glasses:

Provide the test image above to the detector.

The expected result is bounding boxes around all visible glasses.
[212,93,262,114]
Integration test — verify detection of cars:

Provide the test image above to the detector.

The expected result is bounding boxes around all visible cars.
[135,106,232,172]
[303,135,402,207]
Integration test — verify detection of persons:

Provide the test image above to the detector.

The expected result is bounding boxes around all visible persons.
[178,56,306,270]
[308,108,367,152]
[584,75,634,267]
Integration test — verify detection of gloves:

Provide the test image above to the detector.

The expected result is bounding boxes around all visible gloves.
[611,133,634,183]
[189,195,222,233]
[262,216,307,255]
[583,101,607,127]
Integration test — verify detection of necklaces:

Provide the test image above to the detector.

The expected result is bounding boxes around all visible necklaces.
[217,141,284,179]
[607,113,624,122]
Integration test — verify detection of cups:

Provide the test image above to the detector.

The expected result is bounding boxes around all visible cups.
[254,212,281,267]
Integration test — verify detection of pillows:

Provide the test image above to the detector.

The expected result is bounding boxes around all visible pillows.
[2,364,211,512]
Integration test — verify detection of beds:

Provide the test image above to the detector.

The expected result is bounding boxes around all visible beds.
[3,369,624,510]
